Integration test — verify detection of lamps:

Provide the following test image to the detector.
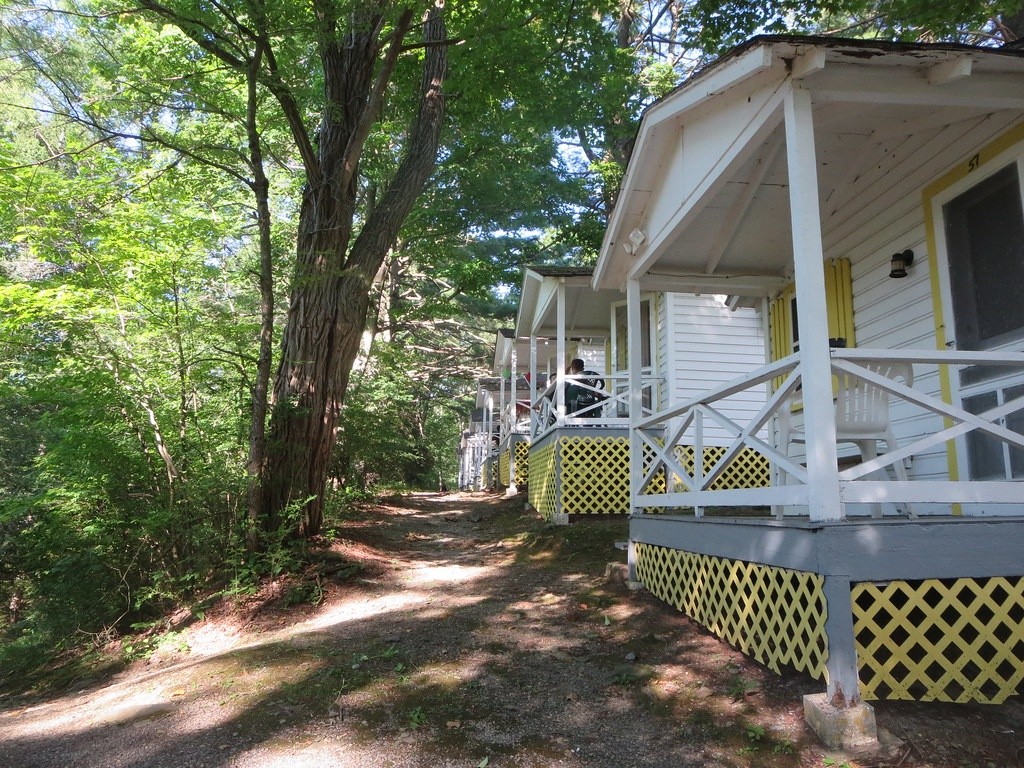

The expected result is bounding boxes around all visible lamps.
[889,249,913,278]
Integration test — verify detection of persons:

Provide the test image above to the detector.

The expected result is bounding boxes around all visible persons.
[565,359,605,419]
[547,373,569,425]
[491,426,500,447]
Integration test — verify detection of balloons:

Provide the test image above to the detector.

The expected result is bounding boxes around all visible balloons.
[503,371,510,379]
[526,373,530,381]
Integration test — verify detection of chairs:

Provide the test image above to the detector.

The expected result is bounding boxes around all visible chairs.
[565,371,605,427]
[767,360,918,520]
